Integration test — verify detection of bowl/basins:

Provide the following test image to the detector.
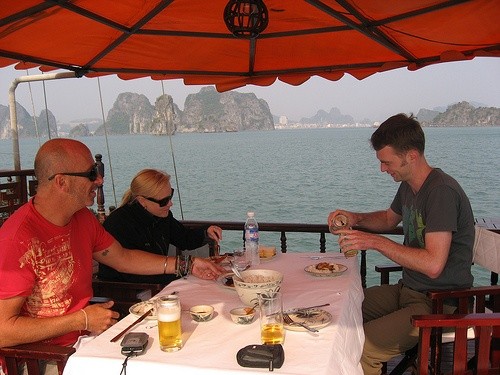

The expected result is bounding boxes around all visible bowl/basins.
[229,306,256,325]
[232,269,284,307]
[189,305,214,322]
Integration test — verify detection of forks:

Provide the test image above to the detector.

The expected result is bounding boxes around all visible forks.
[282,313,318,332]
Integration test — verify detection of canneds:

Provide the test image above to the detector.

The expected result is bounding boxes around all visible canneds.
[329,214,347,235]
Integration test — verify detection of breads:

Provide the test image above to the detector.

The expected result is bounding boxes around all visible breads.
[259,246,276,258]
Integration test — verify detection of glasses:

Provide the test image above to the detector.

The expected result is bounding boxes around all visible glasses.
[135,187,174,207]
[48,162,104,182]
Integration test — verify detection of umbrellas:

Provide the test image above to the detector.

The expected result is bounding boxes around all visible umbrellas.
[0,0,500,199]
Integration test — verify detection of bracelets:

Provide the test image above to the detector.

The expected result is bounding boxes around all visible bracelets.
[163,255,168,275]
[174,254,195,278]
[79,308,88,330]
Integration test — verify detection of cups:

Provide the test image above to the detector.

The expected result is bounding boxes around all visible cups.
[157,301,183,352]
[338,227,358,257]
[259,292,284,345]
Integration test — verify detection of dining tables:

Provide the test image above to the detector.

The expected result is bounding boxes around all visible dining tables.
[63,253,365,375]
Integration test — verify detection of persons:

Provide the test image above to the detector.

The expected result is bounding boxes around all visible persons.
[329,113,476,374]
[0,138,224,375]
[98,168,223,297]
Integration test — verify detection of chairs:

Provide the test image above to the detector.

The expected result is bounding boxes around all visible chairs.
[1,280,160,374]
[374,227,500,375]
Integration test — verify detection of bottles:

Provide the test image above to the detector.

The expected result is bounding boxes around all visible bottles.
[244,212,260,266]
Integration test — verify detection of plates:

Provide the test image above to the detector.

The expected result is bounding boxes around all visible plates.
[304,264,348,278]
[220,272,235,289]
[274,308,333,332]
[260,251,281,261]
[129,300,157,320]
[206,255,233,267]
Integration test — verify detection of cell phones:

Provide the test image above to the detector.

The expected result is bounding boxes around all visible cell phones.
[121,339,148,355]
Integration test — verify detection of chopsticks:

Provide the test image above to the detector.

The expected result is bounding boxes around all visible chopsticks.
[110,291,176,343]
